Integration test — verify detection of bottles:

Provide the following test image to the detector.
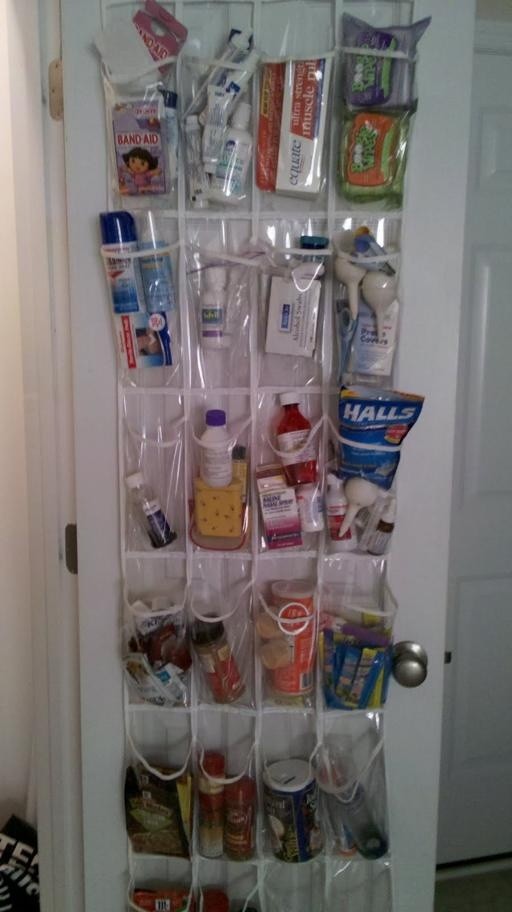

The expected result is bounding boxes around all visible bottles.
[128,595,176,633]
[200,410,232,488]
[135,209,177,316]
[198,267,233,349]
[326,473,358,553]
[274,390,314,486]
[321,760,389,860]
[191,613,247,705]
[231,443,247,503]
[296,484,324,533]
[299,236,326,280]
[196,752,255,861]
[124,471,179,549]
[189,25,262,205]
[366,499,396,556]
[100,208,145,316]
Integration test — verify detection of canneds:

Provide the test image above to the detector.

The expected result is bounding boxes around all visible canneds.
[262,757,324,862]
[266,580,318,696]
